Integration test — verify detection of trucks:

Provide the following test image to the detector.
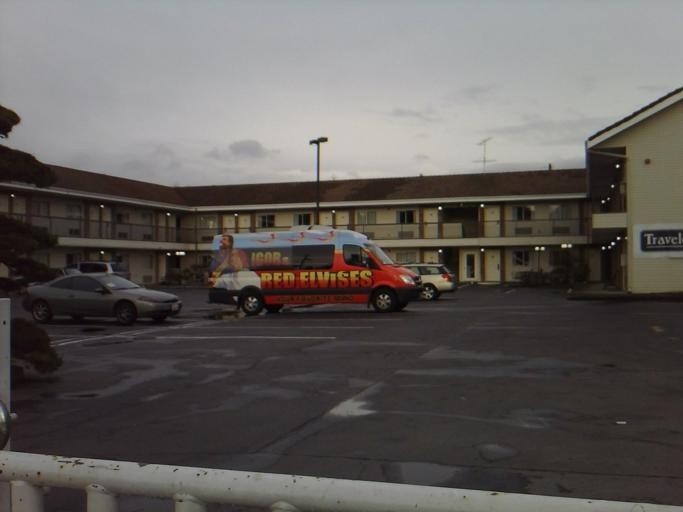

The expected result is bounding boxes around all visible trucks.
[206,225,423,314]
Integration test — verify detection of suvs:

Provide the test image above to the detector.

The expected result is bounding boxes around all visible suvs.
[63,259,132,290]
[397,261,456,300]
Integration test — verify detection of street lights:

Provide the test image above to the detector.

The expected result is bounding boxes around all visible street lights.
[309,135,328,226]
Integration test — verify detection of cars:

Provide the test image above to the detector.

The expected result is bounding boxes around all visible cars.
[22,270,182,327]
